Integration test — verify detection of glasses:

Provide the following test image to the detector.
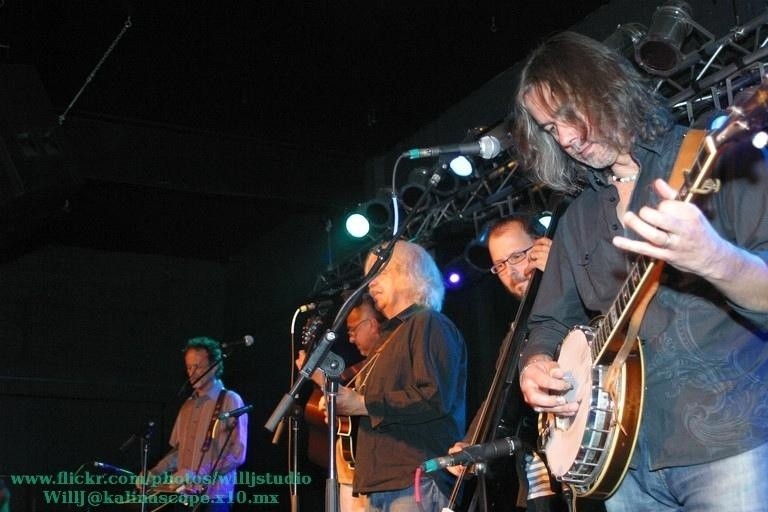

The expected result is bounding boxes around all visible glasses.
[347,319,370,337]
[490,246,534,275]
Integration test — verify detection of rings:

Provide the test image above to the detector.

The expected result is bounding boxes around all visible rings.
[663,233,672,248]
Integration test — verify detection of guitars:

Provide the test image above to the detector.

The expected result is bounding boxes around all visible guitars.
[302,315,359,479]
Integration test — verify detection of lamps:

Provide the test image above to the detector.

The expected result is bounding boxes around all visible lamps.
[345,0,715,241]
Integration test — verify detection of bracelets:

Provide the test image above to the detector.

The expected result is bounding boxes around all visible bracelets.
[519,359,538,380]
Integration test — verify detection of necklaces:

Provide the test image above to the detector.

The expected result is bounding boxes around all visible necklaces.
[611,175,635,184]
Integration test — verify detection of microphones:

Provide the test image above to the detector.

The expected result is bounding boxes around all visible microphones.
[95,461,134,475]
[300,288,364,313]
[401,136,501,162]
[219,404,255,421]
[219,333,255,351]
[422,435,523,474]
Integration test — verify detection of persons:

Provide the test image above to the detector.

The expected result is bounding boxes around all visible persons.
[134,336,249,512]
[324,240,469,512]
[446,213,604,511]
[514,29,768,512]
[295,292,390,512]
